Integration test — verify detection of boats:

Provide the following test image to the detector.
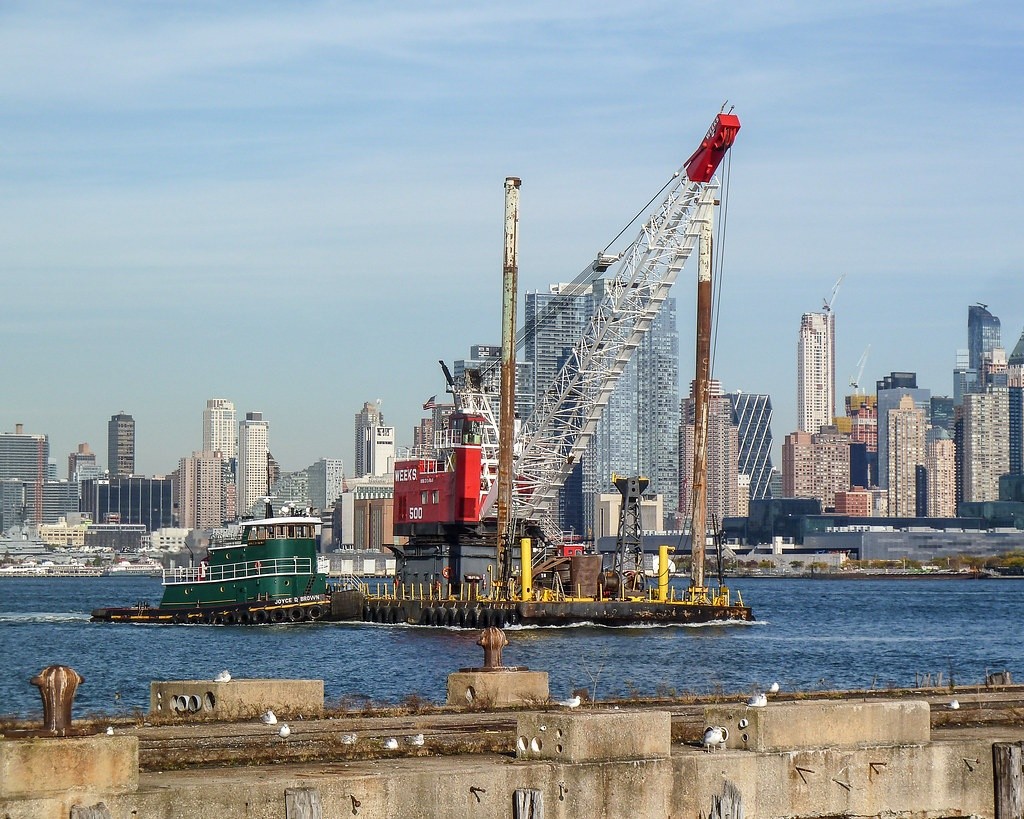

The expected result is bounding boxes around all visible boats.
[98,515,335,623]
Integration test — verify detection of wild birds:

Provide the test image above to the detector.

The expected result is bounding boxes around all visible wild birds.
[556,696,581,711]
[341,733,358,744]
[213,670,231,682]
[699,725,726,754]
[741,682,779,708]
[383,733,425,748]
[279,724,290,744]
[942,699,961,710]
[261,711,278,725]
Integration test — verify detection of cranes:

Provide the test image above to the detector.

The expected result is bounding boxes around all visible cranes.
[388,95,744,615]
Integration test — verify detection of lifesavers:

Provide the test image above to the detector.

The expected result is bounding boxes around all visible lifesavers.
[254,562,261,569]
[442,566,454,578]
